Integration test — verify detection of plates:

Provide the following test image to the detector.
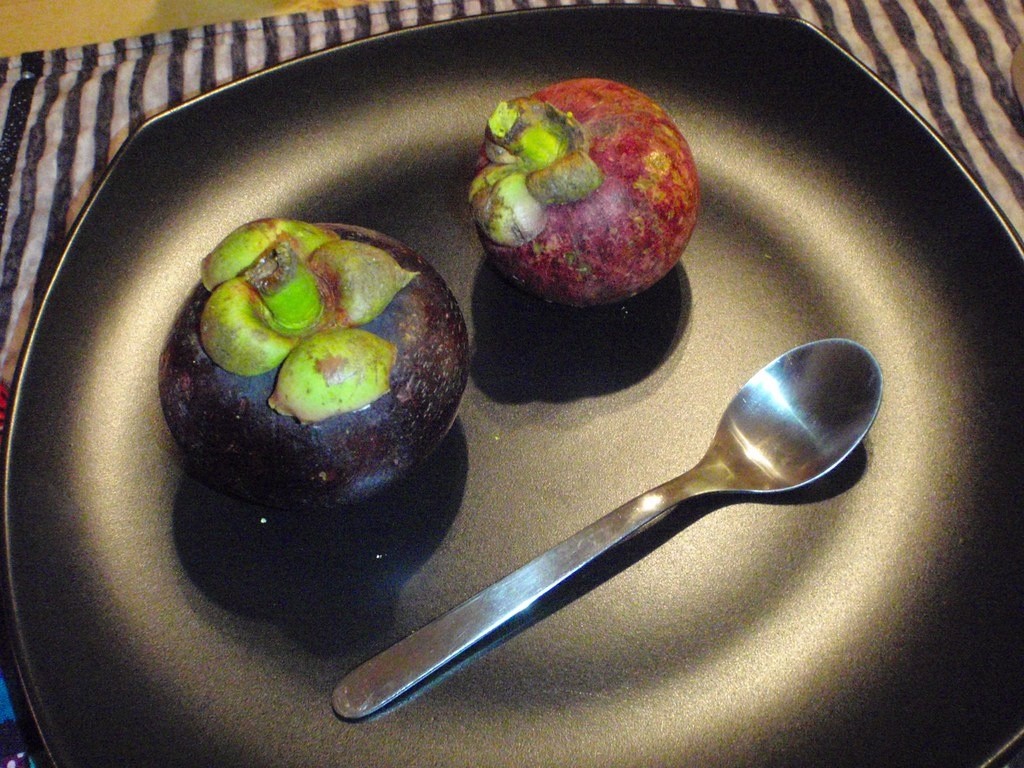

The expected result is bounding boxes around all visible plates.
[5,5,1024,768]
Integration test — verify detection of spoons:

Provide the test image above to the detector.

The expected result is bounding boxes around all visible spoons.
[336,340,880,720]
